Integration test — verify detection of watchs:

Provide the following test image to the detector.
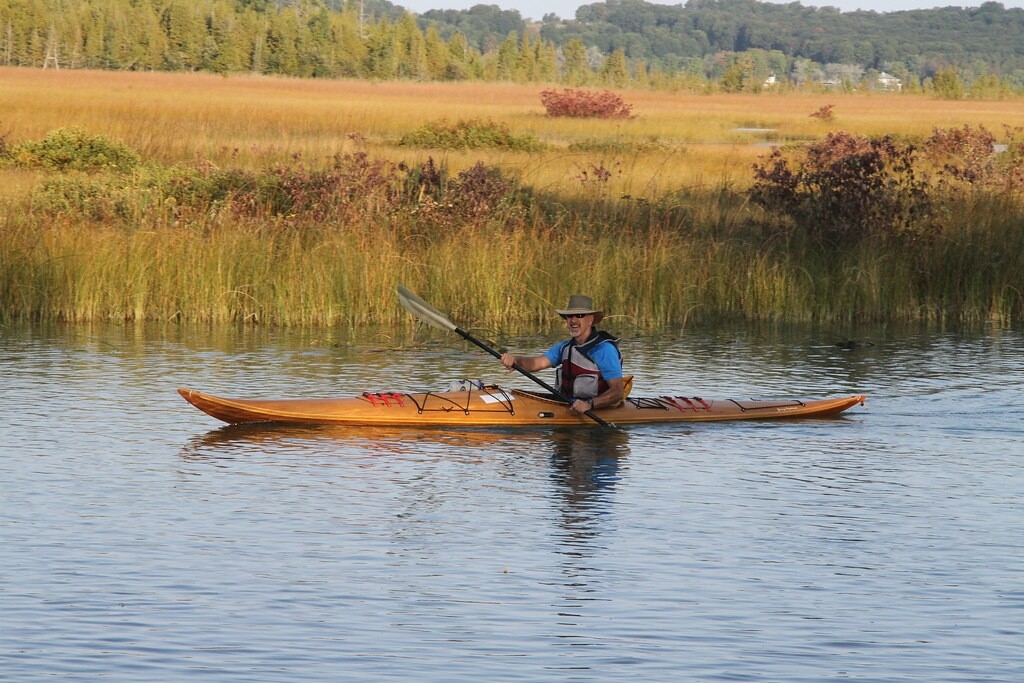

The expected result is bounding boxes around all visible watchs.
[587,398,594,410]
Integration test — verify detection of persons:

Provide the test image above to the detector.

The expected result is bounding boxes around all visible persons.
[501,294,624,416]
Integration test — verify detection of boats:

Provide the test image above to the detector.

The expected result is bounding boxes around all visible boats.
[176,384,865,428]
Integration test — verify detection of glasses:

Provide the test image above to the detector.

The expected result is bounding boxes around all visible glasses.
[567,314,592,318]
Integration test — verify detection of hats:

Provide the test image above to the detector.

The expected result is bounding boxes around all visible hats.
[555,294,603,326]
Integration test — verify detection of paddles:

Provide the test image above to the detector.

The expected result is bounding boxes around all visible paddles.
[396,283,616,433]
